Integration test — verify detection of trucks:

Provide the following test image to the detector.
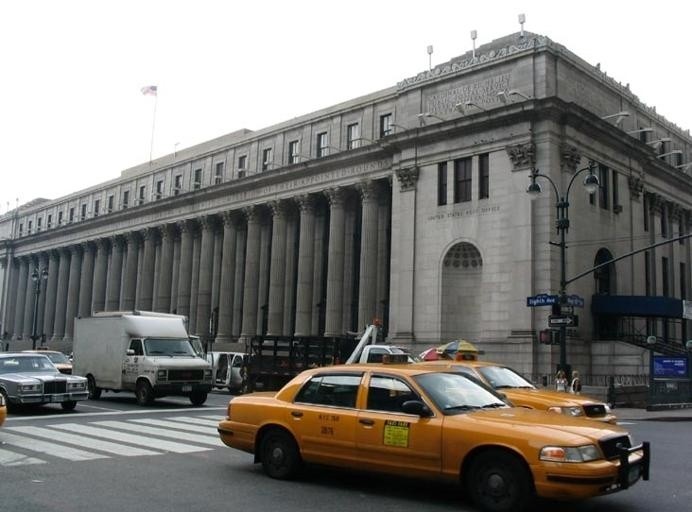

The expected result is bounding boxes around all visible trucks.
[239,324,427,393]
[73,311,215,406]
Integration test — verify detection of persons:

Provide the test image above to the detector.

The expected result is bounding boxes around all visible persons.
[553,369,568,392]
[568,370,583,396]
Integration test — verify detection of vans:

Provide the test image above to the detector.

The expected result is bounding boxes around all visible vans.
[206,352,254,393]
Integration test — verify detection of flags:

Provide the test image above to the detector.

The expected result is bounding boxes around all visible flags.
[140,85,157,96]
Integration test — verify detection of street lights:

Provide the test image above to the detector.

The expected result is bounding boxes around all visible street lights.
[31,266,50,350]
[525,162,599,383]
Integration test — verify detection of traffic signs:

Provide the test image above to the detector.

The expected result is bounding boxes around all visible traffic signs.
[527,293,584,327]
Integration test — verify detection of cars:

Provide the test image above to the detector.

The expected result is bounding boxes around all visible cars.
[217,354,650,512]
[0,347,90,427]
[412,348,618,425]
